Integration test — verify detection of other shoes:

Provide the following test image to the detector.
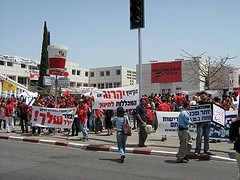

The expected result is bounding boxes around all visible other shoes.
[139,145,147,147]
[195,151,199,155]
[121,156,124,163]
[184,157,189,161]
[178,159,188,163]
[205,152,211,154]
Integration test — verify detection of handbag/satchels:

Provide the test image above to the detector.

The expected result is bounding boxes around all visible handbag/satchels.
[122,118,132,136]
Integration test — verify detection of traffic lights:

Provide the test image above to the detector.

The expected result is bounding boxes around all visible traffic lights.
[129,0,145,30]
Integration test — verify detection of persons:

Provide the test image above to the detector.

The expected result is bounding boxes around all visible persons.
[75,99,90,142]
[229,104,240,179]
[0,94,26,133]
[105,93,187,135]
[110,106,132,164]
[194,91,215,156]
[135,98,148,147]
[17,100,31,134]
[27,94,103,137]
[189,89,240,143]
[176,101,197,163]
